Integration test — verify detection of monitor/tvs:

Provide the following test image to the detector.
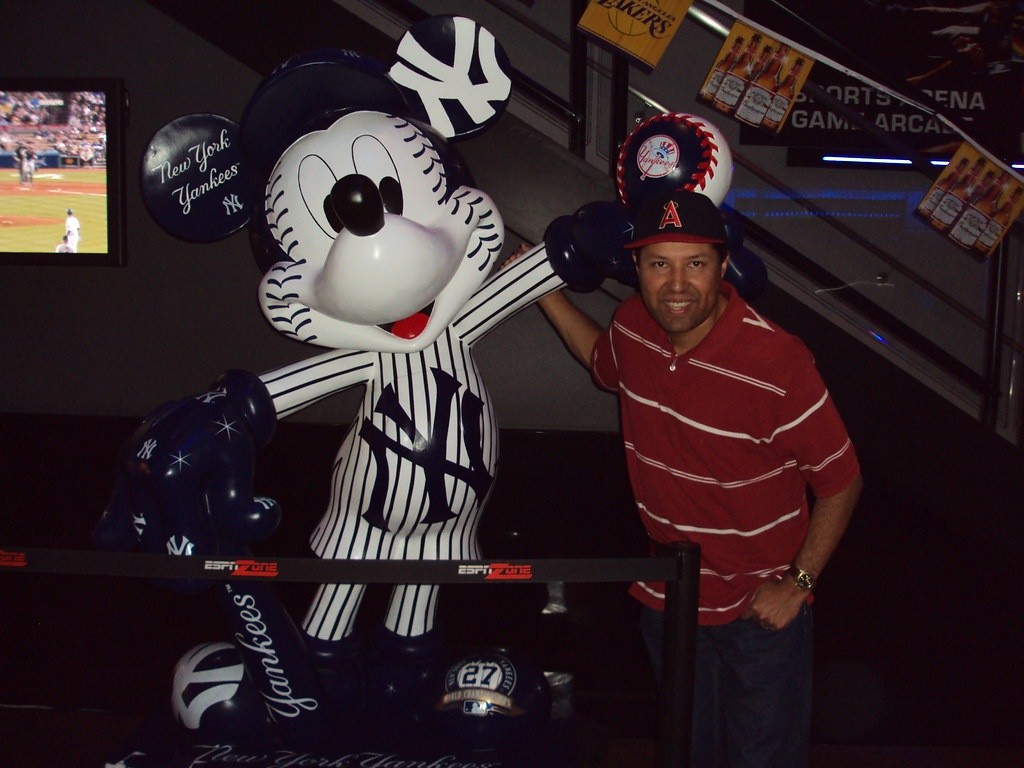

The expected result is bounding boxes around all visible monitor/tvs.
[0,75,128,267]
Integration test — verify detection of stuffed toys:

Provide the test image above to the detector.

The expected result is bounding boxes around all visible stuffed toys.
[91,11,769,756]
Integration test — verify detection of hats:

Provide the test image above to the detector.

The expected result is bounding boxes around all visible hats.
[623,189,727,249]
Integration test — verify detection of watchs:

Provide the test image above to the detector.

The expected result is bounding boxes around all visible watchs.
[788,565,819,590]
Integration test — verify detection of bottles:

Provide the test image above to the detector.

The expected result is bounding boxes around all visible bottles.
[750,44,774,84]
[540,575,577,688]
[974,186,1024,254]
[702,36,745,101]
[945,171,1014,253]
[761,57,805,132]
[734,42,791,128]
[711,33,763,114]
[919,157,970,219]
[948,171,995,233]
[927,157,987,234]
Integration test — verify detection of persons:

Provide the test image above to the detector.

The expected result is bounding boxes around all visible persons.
[499,190,865,767]
[56,208,81,253]
[0,91,106,182]
